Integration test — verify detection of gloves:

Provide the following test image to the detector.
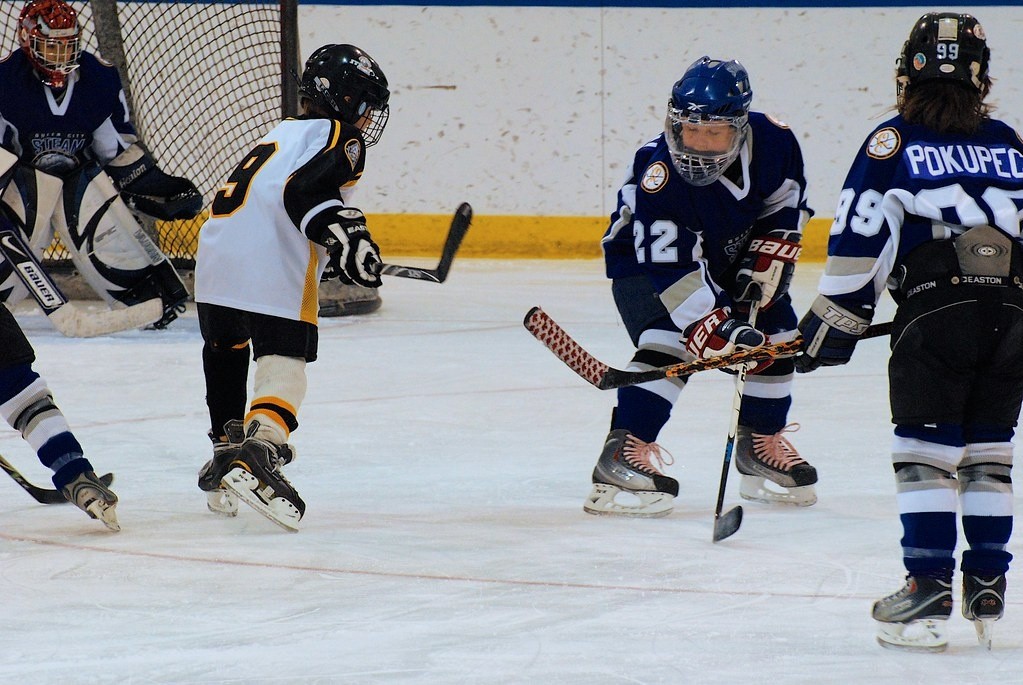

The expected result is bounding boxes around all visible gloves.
[791,312,856,374]
[321,208,384,289]
[678,309,774,376]
[733,237,803,312]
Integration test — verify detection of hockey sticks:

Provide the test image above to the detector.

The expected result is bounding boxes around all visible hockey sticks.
[379,201,477,285]
[0,205,165,339]
[1,457,113,503]
[522,305,893,394]
[712,298,761,544]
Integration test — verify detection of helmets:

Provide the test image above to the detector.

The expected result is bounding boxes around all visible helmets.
[664,55,752,187]
[896,10,990,115]
[297,42,391,148]
[17,0,81,88]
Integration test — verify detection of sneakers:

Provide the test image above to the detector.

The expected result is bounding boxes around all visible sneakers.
[52,458,123,533]
[220,419,306,534]
[961,570,1007,649]
[734,422,818,507]
[871,574,955,654]
[197,420,245,519]
[583,428,680,518]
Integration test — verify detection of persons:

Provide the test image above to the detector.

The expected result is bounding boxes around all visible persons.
[591,55,819,498]
[193,42,392,523]
[0,223,121,521]
[791,11,1023,626]
[0,0,205,332]
